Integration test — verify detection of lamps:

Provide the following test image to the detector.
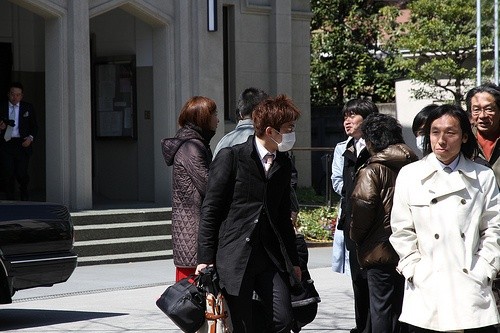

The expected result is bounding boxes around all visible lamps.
[207,0,217,32]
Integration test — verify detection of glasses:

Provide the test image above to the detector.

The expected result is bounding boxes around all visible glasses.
[279,124,296,133]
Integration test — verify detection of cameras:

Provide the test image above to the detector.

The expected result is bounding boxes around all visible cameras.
[0,118,16,127]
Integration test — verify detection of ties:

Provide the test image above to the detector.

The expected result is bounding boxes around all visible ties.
[4,105,17,141]
[265,152,275,174]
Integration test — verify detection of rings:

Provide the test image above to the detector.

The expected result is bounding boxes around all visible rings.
[25,142,27,145]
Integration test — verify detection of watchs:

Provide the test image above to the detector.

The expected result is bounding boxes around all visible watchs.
[29,135,34,141]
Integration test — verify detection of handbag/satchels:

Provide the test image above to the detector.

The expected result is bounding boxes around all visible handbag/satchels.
[156,274,205,333]
[196,268,234,333]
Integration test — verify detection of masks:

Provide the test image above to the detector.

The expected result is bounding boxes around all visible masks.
[416,135,427,152]
[270,129,296,152]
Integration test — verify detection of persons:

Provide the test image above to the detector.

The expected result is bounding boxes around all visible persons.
[0,84,35,204]
[331,132,353,275]
[350,111,422,333]
[411,104,439,154]
[196,94,301,333]
[337,97,380,332]
[389,102,500,333]
[159,96,220,286]
[465,81,500,319]
[212,87,268,165]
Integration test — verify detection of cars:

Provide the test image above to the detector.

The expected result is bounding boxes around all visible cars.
[0,200,79,302]
